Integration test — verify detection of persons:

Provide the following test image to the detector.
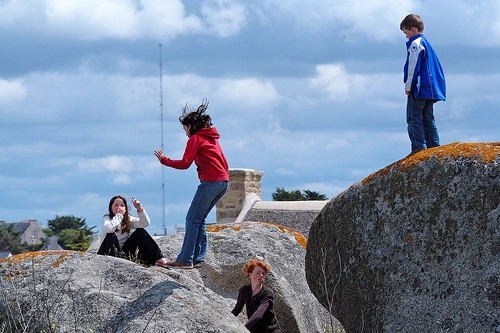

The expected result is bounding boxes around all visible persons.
[85,195,172,268]
[154,97,230,268]
[400,14,446,155]
[230,259,283,333]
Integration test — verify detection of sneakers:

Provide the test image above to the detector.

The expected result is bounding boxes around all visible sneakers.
[155,257,203,269]
